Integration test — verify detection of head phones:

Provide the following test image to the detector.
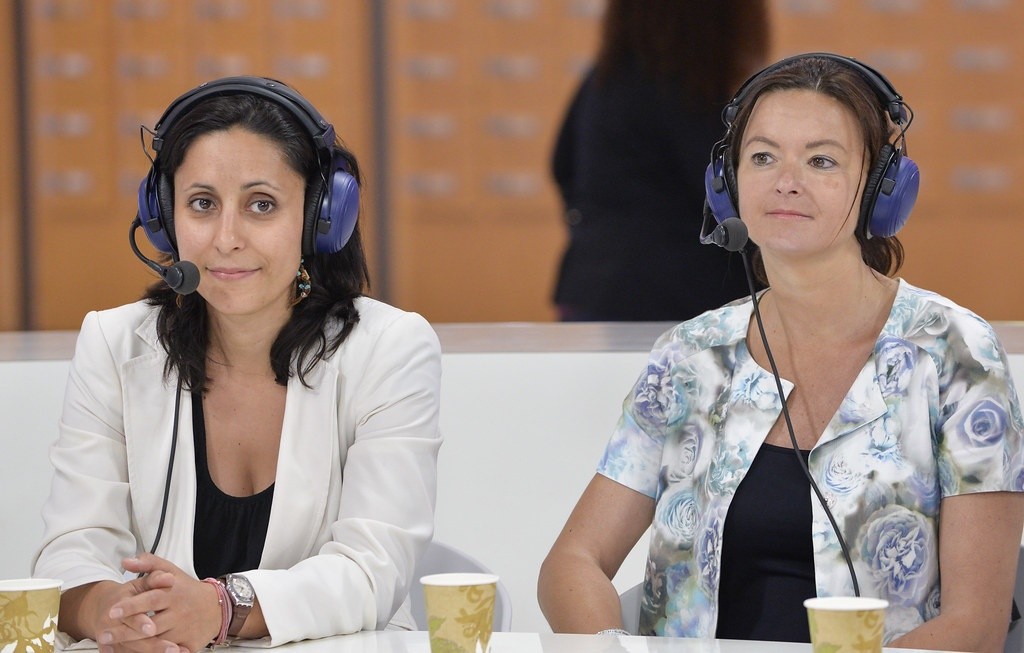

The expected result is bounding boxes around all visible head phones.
[706,53,919,240]
[136,76,362,257]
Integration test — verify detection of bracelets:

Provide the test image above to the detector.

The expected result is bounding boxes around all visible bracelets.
[596,629,633,638]
[203,576,230,649]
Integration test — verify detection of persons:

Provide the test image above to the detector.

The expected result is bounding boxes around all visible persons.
[34,74,443,653]
[550,0,782,320]
[535,49,1021,651]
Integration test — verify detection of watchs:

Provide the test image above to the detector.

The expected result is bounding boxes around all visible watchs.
[217,570,256,638]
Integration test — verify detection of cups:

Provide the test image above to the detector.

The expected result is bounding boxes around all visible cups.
[803,596,889,653]
[420,572,498,653]
[1,579,61,653]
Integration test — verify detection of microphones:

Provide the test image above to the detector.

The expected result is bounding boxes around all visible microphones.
[699,200,750,252]
[129,220,200,294]
